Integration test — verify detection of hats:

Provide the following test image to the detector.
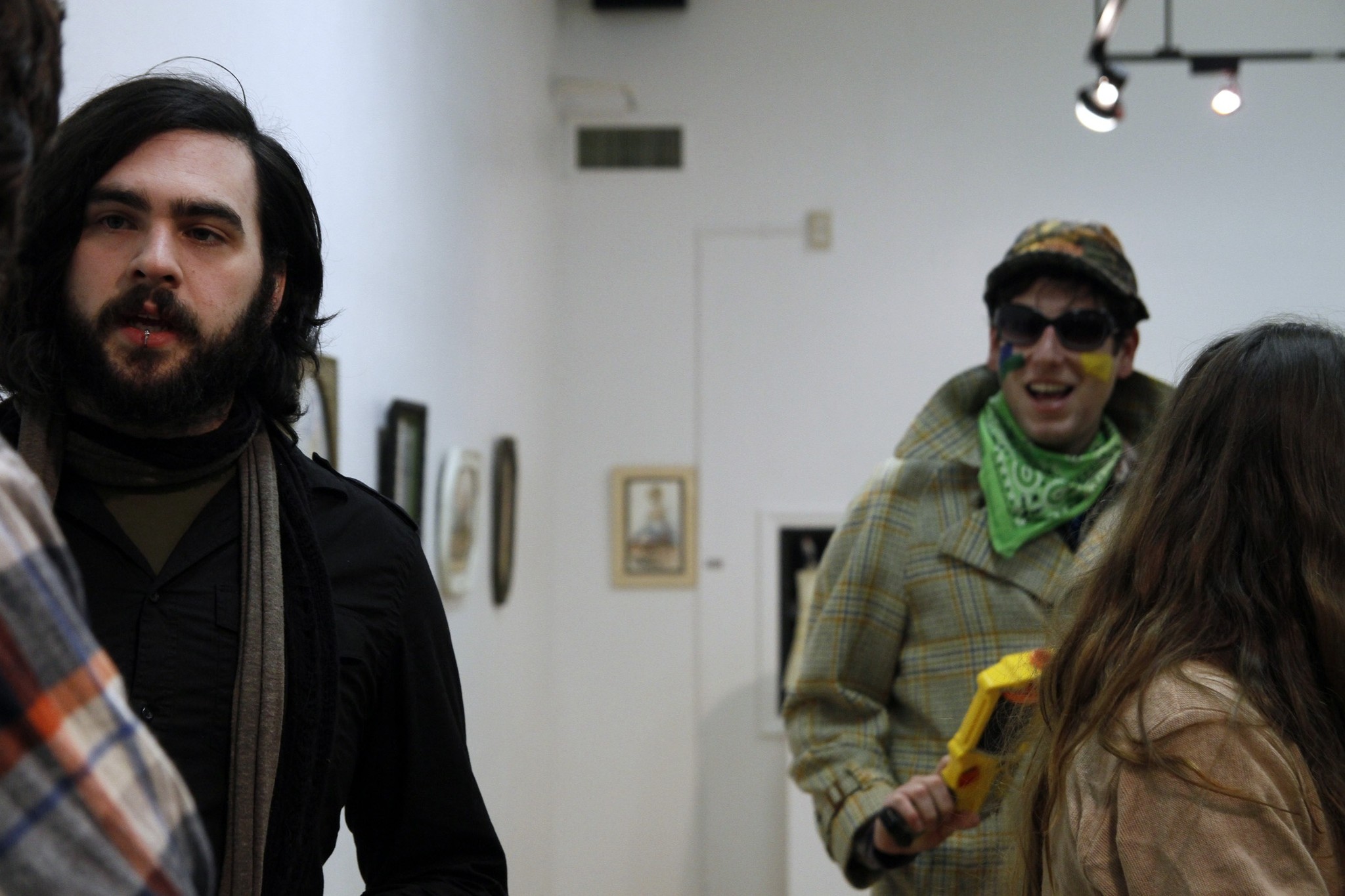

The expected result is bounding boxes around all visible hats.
[984,219,1150,323]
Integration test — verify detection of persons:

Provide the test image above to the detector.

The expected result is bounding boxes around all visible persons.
[0,54,508,896]
[780,219,1177,896]
[1010,323,1344,895]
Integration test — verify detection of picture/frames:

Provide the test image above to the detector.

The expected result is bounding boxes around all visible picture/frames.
[610,465,697,589]
[385,402,518,604]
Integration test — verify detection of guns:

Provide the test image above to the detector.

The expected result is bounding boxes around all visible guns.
[880,646,1054,848]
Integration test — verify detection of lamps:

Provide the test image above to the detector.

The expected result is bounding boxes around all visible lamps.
[1074,67,1129,131]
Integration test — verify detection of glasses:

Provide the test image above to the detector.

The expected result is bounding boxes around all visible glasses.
[997,304,1122,352]
